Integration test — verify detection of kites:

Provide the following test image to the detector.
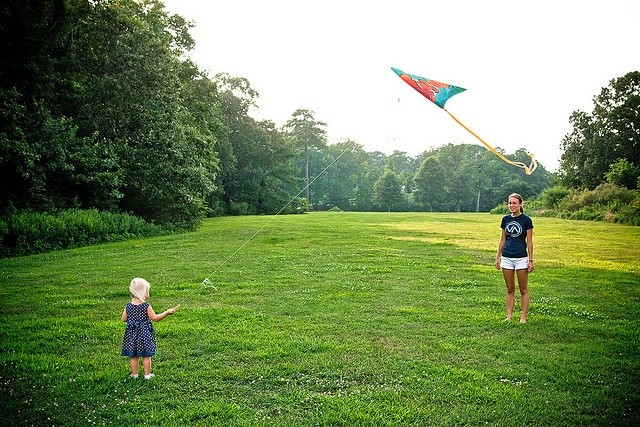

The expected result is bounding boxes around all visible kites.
[391,65,537,174]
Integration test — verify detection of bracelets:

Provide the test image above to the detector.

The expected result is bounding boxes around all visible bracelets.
[529,260,534,262]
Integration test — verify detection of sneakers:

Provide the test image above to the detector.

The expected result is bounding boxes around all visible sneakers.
[144,373,155,381]
[130,374,138,379]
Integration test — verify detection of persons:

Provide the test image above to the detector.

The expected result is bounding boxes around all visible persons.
[122,278,175,380]
[495,193,534,323]
